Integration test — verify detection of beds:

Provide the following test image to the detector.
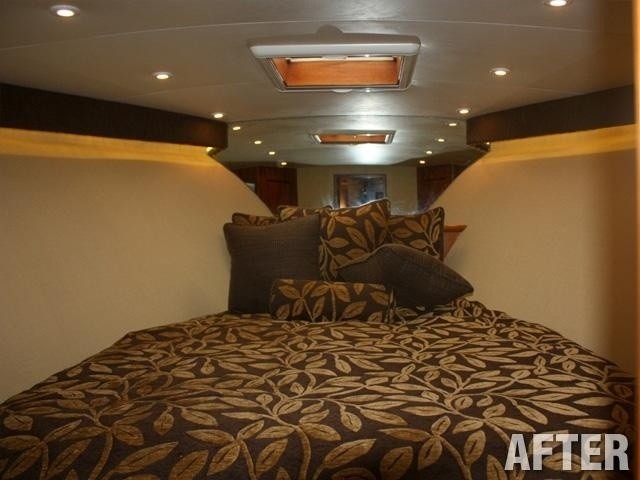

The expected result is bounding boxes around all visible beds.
[1,312,640,479]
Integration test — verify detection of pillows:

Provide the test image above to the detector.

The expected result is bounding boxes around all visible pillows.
[216,199,476,320]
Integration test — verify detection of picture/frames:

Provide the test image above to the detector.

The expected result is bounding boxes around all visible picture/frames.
[332,173,387,209]
